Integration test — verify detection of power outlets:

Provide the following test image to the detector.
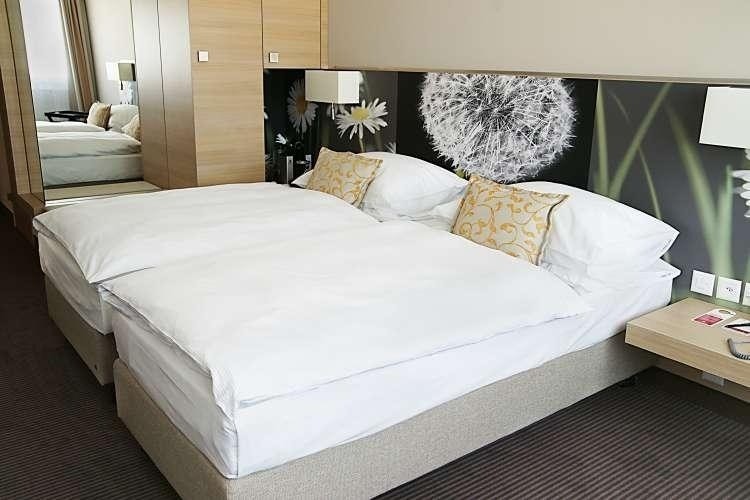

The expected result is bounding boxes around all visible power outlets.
[716,277,743,304]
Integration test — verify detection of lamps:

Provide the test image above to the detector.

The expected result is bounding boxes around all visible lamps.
[700,86,750,151]
[106,61,136,92]
[304,70,363,121]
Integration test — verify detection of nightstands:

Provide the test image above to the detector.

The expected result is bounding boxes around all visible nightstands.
[45,111,89,123]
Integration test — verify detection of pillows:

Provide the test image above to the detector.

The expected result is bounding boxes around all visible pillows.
[473,183,682,298]
[306,146,385,206]
[451,175,560,269]
[289,152,461,224]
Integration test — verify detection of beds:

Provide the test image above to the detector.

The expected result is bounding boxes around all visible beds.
[37,144,467,388]
[104,175,686,498]
[35,102,140,190]
